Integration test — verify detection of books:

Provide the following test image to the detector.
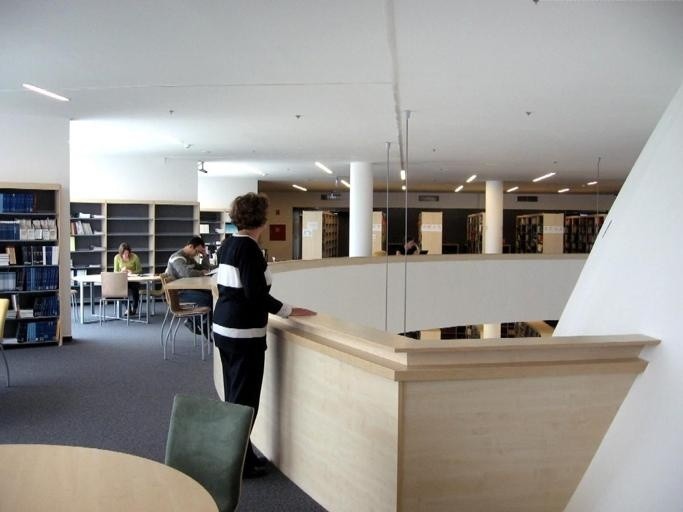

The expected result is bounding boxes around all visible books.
[515,217,543,253]
[465,215,482,252]
[0,190,59,344]
[69,212,101,285]
[562,216,603,252]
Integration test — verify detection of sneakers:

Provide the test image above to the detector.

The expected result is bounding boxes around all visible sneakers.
[124,307,136,316]
[203,323,213,342]
[183,319,201,335]
[252,458,268,477]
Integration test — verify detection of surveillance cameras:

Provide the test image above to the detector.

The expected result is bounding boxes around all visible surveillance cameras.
[198,168,207,173]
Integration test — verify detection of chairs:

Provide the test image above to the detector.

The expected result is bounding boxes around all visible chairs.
[96,271,134,328]
[164,390,255,511]
[137,273,167,320]
[158,271,212,362]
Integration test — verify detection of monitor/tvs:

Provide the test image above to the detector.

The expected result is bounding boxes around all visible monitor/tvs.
[419,250,429,255]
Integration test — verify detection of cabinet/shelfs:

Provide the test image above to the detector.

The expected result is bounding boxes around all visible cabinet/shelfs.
[298,209,341,259]
[466,210,485,254]
[372,209,391,254]
[515,210,607,256]
[71,200,227,303]
[0,180,69,351]
[416,211,443,254]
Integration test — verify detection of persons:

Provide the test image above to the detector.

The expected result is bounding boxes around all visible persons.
[210,192,317,476]
[113,242,142,316]
[164,235,214,342]
[395,237,420,256]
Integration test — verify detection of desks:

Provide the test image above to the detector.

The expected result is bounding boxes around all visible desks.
[1,442,220,511]
[72,272,155,326]
